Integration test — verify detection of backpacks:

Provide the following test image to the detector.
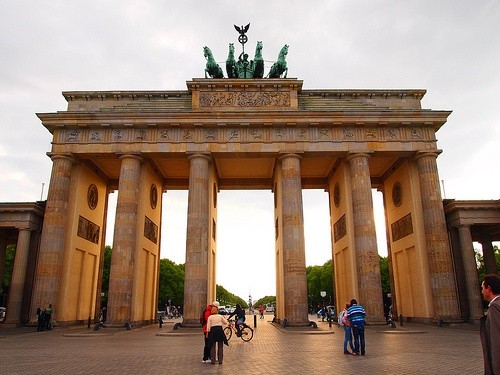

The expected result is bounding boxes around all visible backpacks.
[200,309,206,325]
[338,311,345,326]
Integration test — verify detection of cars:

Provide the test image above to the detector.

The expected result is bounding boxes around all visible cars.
[265,303,274,312]
[218,304,236,315]
[316,306,335,319]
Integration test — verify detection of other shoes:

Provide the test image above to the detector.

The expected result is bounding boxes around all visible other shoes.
[202,357,211,363]
[352,352,359,356]
[344,350,351,354]
[219,362,222,364]
[361,353,364,355]
[211,362,215,365]
[237,333,242,337]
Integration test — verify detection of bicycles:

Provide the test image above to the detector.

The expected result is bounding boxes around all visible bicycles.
[222,319,253,342]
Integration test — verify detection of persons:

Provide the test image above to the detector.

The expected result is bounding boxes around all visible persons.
[479,274,500,375]
[101,306,106,321]
[202,301,219,363]
[168,305,182,319]
[227,304,245,337]
[383,304,389,325]
[342,303,360,354]
[321,305,332,322]
[347,299,366,355]
[259,305,264,319]
[206,307,229,365]
[43,304,53,331]
[238,52,249,67]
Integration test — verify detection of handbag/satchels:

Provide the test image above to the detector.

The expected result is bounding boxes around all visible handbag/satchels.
[238,310,245,317]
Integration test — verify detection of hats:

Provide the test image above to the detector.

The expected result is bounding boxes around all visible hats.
[213,301,219,306]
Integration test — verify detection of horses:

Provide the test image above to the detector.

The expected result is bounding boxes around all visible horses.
[226,42,239,78]
[252,40,264,78]
[269,44,290,78]
[202,46,225,79]
